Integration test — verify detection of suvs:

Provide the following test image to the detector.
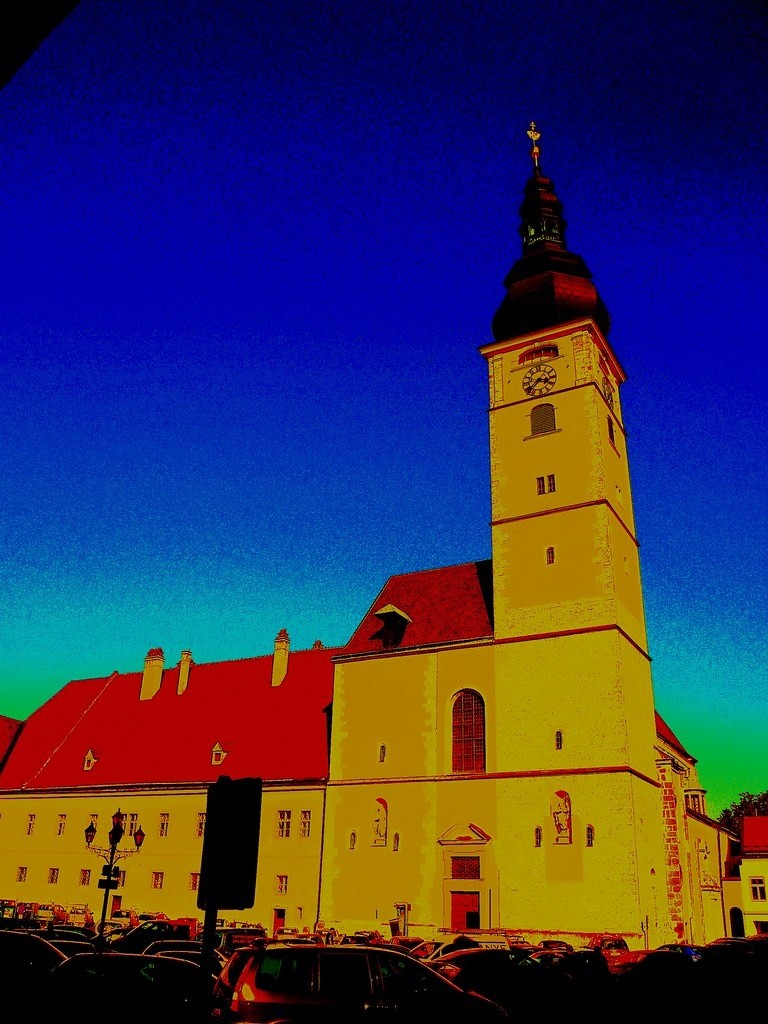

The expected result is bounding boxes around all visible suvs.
[213,944,508,1023]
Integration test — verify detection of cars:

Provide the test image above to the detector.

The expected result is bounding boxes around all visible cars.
[0,900,768,1024]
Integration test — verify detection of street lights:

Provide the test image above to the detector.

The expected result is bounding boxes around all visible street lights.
[84,808,146,934]
